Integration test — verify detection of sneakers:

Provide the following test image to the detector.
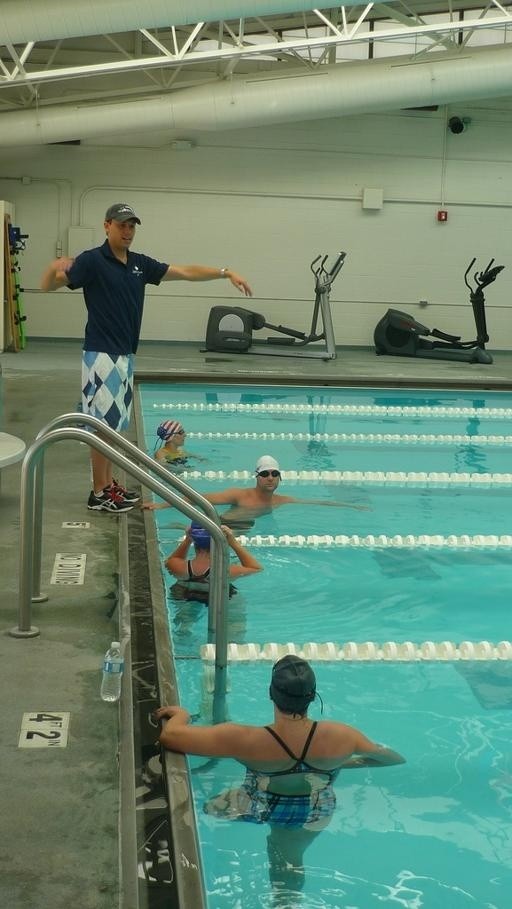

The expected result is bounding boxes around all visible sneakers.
[87,476,141,513]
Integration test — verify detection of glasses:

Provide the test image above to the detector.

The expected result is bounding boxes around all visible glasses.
[259,470,281,477]
[166,429,184,435]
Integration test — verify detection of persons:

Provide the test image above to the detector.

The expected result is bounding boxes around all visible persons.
[40,202,252,517]
[140,453,369,536]
[153,418,205,471]
[152,653,409,908]
[161,515,261,643]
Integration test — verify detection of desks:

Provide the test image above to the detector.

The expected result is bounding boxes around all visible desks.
[1,430,27,473]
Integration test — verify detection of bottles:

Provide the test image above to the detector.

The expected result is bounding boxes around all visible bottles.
[100,641,125,702]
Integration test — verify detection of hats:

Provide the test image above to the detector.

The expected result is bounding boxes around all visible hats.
[105,203,141,225]
[157,419,182,443]
[188,519,211,548]
[268,651,317,714]
[253,455,280,476]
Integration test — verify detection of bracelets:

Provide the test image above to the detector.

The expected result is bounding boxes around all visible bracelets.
[220,267,228,279]
